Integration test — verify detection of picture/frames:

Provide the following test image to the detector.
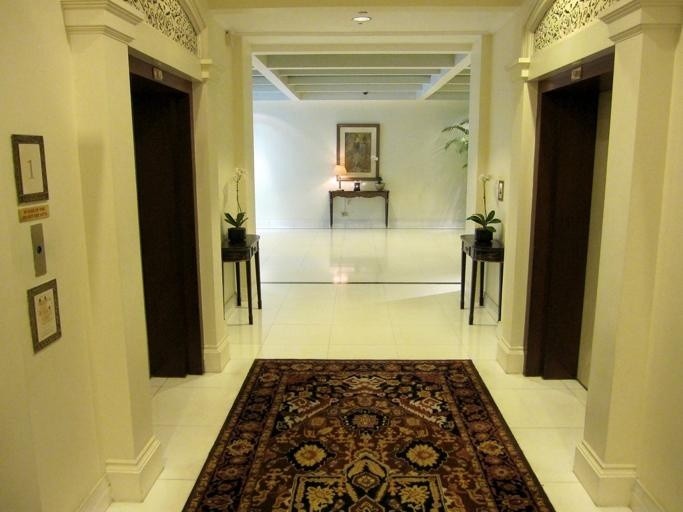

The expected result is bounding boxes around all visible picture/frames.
[336,124,379,181]
[25,278,61,354]
[11,135,49,204]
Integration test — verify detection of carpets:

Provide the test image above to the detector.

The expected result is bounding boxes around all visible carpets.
[181,359,557,512]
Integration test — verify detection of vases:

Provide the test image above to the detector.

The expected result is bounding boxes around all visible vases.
[474,227,493,242]
[227,228,245,239]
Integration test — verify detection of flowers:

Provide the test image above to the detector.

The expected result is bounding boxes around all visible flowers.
[224,168,247,227]
[464,174,501,233]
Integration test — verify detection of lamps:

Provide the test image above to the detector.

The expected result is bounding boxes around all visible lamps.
[333,165,346,189]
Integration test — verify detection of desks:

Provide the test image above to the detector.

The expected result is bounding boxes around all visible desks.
[223,234,260,326]
[329,190,389,228]
[460,234,503,326]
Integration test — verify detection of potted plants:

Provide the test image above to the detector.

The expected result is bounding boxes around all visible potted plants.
[374,177,385,190]
